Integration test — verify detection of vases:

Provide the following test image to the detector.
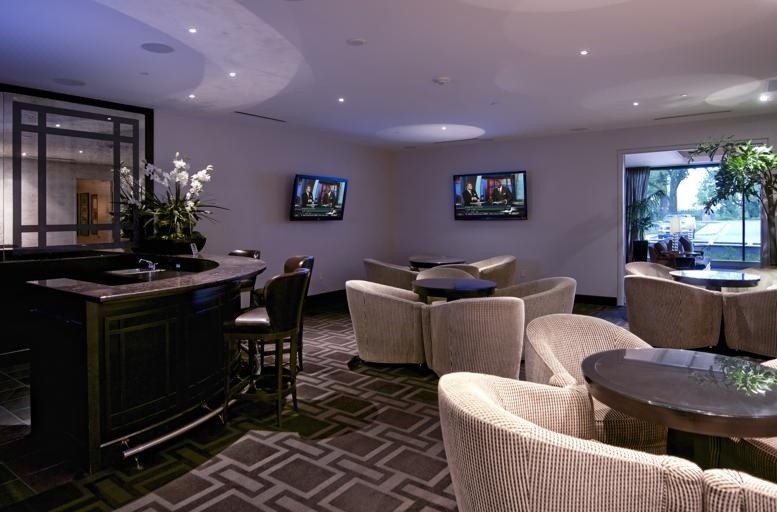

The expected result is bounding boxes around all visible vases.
[131,231,206,255]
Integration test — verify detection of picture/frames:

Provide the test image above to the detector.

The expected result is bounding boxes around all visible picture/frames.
[80,193,89,236]
[91,194,98,235]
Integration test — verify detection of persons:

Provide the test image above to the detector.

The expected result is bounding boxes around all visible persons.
[301,184,314,205]
[491,179,513,205]
[462,181,480,208]
[322,185,336,208]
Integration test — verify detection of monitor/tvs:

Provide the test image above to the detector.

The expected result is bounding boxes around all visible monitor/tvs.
[453,170,527,220]
[290,174,348,221]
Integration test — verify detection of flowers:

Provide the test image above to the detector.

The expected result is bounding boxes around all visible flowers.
[109,151,231,241]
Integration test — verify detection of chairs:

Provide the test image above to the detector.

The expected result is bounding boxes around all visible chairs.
[253,255,315,370]
[222,268,312,426]
[227,249,261,365]
